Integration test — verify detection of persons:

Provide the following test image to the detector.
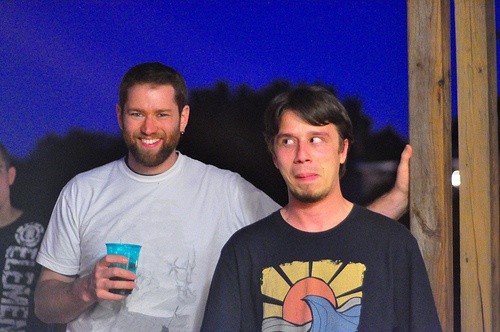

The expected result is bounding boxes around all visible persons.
[201,84,446,331]
[0,143,64,331]
[26,62,413,332]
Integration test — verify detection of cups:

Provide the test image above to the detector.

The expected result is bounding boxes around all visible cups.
[105,242,142,295]
